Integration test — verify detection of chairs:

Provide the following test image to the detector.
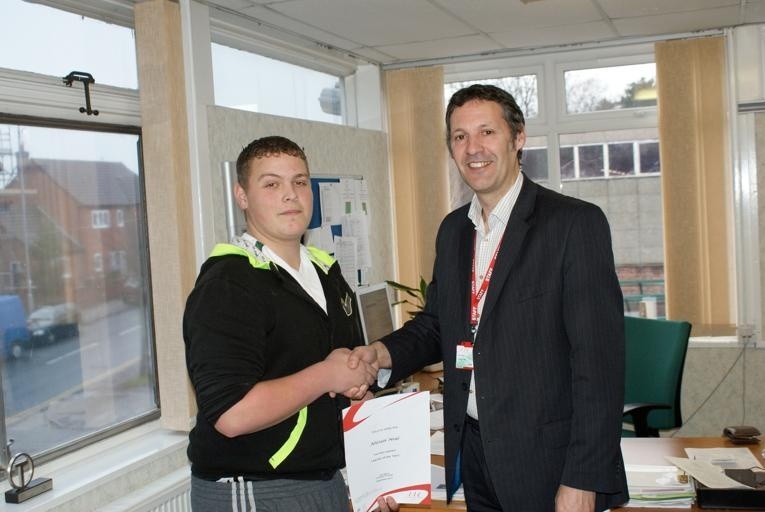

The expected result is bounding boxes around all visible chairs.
[621,315,692,438]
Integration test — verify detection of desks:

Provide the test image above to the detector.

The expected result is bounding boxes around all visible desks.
[396,434,764,512]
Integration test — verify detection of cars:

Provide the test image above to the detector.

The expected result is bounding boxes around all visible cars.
[26,303,79,345]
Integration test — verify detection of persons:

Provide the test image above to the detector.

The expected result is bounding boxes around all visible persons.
[329,83,630,512]
[182,135,399,512]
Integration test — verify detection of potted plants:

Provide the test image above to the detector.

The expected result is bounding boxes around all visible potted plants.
[384,275,444,373]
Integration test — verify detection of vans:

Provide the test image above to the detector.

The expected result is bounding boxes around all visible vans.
[0,294,31,362]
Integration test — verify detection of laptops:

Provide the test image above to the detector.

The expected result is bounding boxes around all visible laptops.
[355,281,397,346]
